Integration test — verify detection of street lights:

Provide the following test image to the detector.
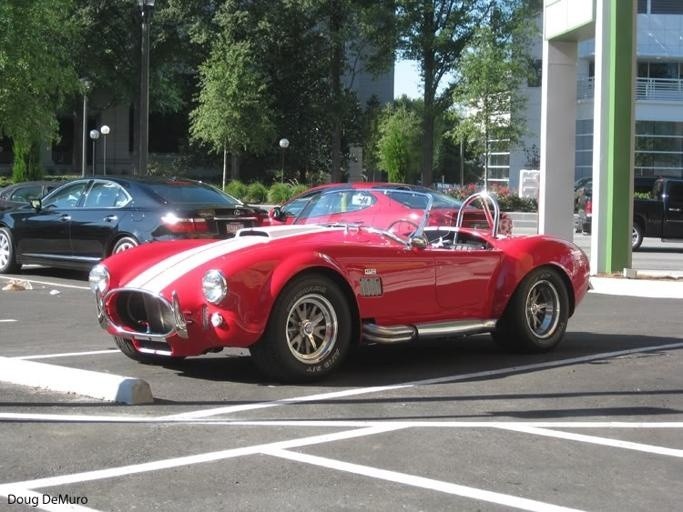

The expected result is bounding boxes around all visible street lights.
[77,77,111,180]
[279,138,290,184]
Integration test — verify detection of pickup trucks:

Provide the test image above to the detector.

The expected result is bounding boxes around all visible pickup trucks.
[582,178,683,250]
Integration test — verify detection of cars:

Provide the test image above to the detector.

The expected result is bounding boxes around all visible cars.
[0,176,591,383]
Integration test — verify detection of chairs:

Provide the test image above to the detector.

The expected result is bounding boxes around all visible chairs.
[97,191,114,206]
